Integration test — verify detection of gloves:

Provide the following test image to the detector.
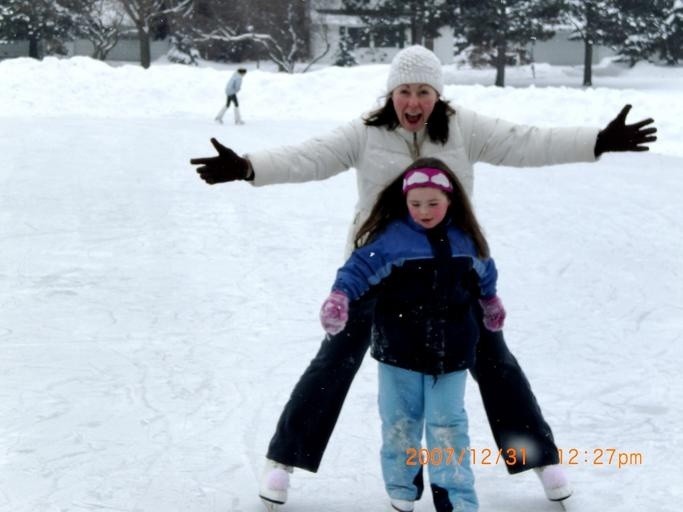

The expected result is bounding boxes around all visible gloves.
[479,295,507,332]
[188,137,249,185]
[319,289,350,336]
[594,104,659,159]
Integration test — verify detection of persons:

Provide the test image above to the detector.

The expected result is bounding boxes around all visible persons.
[215,69,246,125]
[322,157,506,511]
[188,45,658,505]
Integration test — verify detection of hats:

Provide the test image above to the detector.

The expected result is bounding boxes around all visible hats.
[385,43,447,99]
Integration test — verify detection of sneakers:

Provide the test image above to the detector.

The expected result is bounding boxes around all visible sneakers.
[391,497,414,512]
[532,463,574,502]
[257,456,295,505]
